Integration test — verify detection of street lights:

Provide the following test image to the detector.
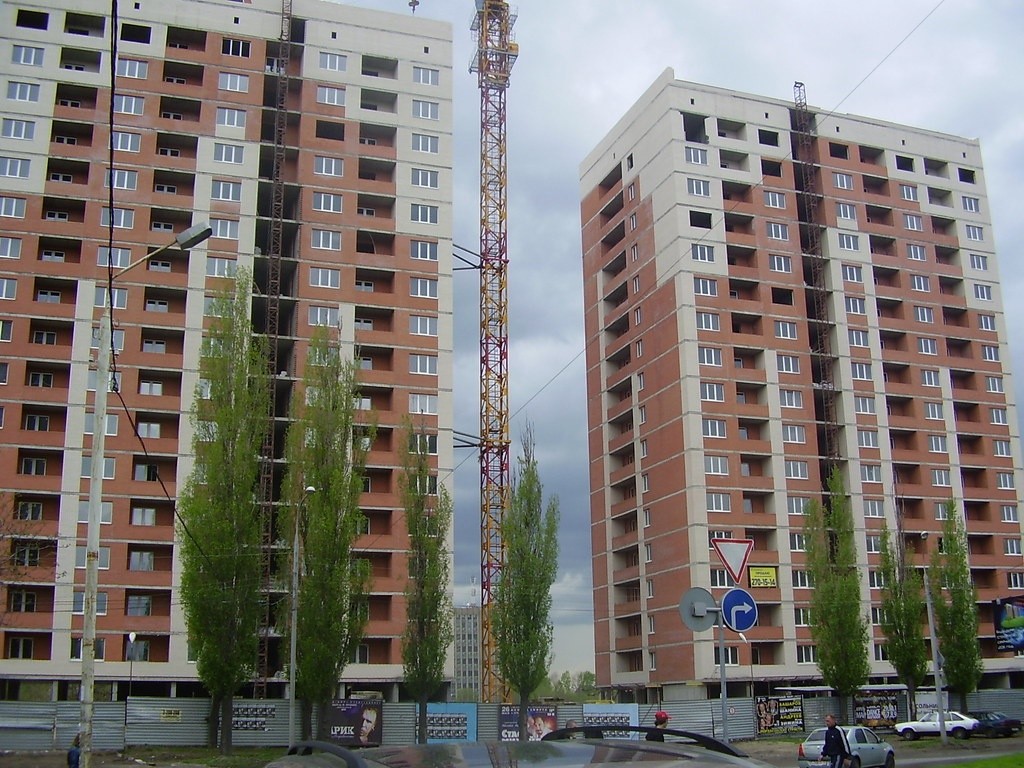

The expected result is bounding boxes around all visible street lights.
[288,487,318,748]
[76,224,216,767]
[129,632,136,696]
[921,531,948,745]
[739,633,755,699]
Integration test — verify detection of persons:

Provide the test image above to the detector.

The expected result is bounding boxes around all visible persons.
[355,705,378,743]
[818,715,851,768]
[533,715,553,738]
[67,732,79,768]
[755,699,778,731]
[645,712,671,742]
[566,719,577,739]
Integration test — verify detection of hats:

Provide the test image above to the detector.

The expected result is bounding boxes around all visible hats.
[655,711,672,720]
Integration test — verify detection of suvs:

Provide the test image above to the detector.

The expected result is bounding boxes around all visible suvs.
[262,722,777,768]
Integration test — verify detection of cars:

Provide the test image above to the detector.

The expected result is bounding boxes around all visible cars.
[894,709,980,741]
[966,711,1021,738]
[796,725,895,768]
